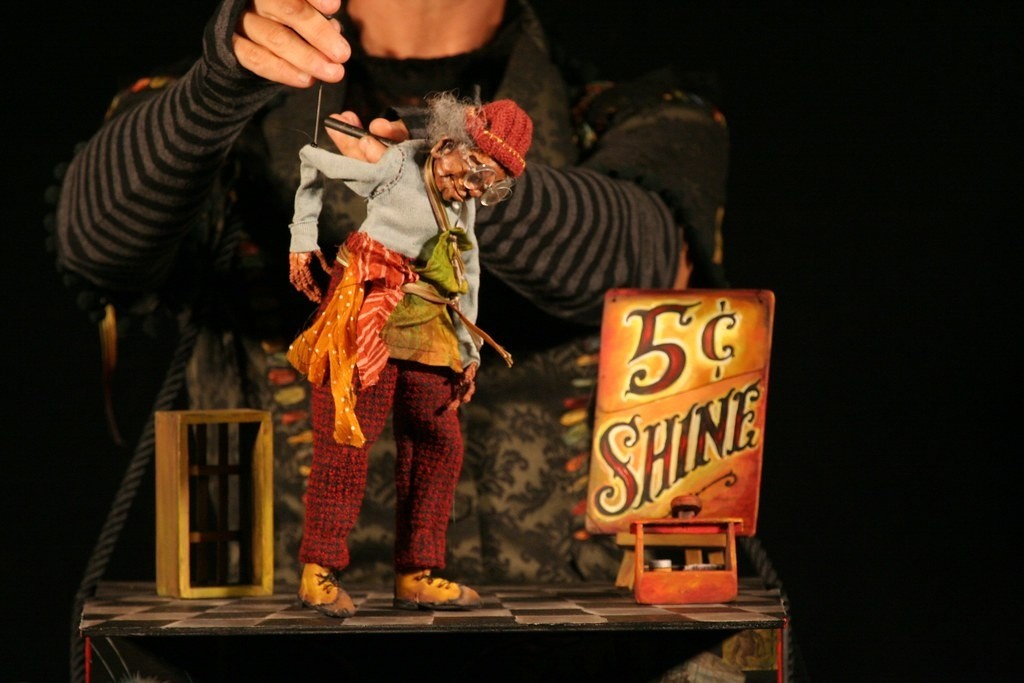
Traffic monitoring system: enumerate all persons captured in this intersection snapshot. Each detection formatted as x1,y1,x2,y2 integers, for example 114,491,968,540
56,0,728,586
289,92,534,614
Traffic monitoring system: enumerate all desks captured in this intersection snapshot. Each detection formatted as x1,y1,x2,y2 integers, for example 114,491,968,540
79,577,787,683
